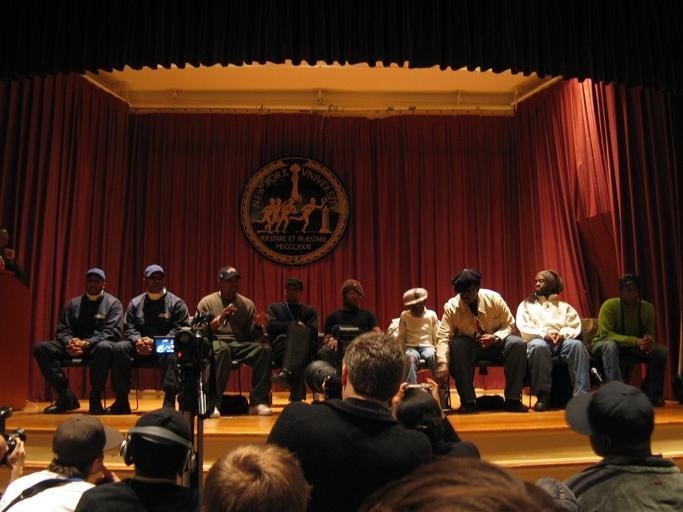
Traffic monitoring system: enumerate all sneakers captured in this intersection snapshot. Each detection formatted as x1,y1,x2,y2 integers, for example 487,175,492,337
249,404,273,416
207,404,220,418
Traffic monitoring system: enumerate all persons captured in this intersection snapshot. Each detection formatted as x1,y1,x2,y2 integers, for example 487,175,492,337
101,264,190,414
434,268,529,414
263,276,318,402
323,278,382,360
0,228,22,282
515,269,592,412
266,330,433,512
32,268,123,413
198,440,313,511
590,272,669,406
193,265,273,418
0,412,124,512
0,435,25,498
390,378,481,460
396,287,451,412
562,380,682,511
73,407,196,512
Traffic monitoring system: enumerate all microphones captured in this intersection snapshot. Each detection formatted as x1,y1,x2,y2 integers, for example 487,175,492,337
222,303,235,326
591,368,604,385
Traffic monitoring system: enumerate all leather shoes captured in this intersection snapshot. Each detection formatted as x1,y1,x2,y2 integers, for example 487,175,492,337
270,368,298,387
43,396,133,415
646,393,664,407
456,400,547,412
162,393,175,412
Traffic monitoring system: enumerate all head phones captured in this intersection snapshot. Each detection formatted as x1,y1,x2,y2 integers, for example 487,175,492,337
118,426,196,477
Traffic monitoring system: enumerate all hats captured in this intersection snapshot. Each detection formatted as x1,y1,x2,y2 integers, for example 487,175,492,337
566,381,654,446
285,279,303,291
403,267,484,306
86,268,105,280
537,267,565,294
217,266,241,281
144,264,166,279
619,274,641,291
340,279,364,296
51,415,106,467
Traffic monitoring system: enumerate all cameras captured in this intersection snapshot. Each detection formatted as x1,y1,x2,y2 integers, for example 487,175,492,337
405,384,430,397
0,407,26,464
